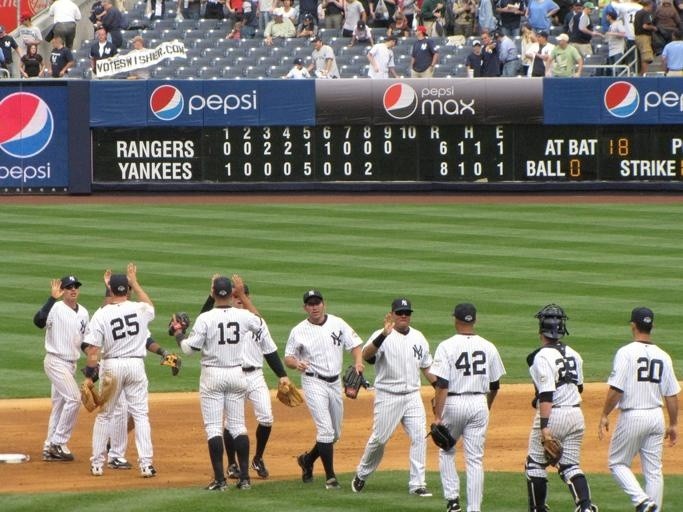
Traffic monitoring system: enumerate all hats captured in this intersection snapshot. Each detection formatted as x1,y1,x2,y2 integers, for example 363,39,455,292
629,307,653,326
417,26,430,37
522,22,570,41
584,1,595,9
391,297,413,312
214,276,250,299
60,275,81,289
472,40,482,46
302,13,314,20
357,20,365,32
271,8,284,16
309,35,321,43
110,274,129,294
293,57,306,67
303,289,323,303
451,303,476,322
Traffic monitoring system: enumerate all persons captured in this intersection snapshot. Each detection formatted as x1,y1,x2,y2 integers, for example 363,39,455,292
102,267,181,470
429,303,507,512
201,272,291,479
351,298,437,497
284,289,365,489
171,273,266,492
598,307,682,512
0,0,683,79
82,262,156,478
525,304,599,512
33,274,100,461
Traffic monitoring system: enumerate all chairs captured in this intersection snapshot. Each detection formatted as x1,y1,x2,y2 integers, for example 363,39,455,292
64,0,608,80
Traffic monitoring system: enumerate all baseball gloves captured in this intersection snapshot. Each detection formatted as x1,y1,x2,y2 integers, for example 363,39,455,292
541,435,563,467
276,380,304,407
343,366,368,397
160,352,180,374
430,422,456,450
80,363,102,412
169,313,190,336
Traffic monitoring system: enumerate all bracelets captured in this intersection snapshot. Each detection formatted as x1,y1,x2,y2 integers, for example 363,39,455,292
85,366,94,377
541,418,548,430
157,347,166,355
373,334,385,348
431,382,436,388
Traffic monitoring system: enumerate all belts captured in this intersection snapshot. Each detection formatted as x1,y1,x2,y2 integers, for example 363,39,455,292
552,403,581,408
242,366,261,373
447,392,481,396
306,372,338,383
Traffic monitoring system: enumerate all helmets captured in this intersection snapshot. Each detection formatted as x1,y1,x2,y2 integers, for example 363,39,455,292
535,302,569,340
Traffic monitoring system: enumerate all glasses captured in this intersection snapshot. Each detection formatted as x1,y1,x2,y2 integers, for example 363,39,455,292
63,284,79,290
394,310,411,317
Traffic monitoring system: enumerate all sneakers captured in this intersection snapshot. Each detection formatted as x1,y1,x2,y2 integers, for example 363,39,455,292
414,488,432,496
90,467,103,476
141,465,157,478
325,480,342,490
298,451,314,483
205,455,267,491
107,457,132,469
351,473,365,492
41,442,73,461
447,500,462,512
